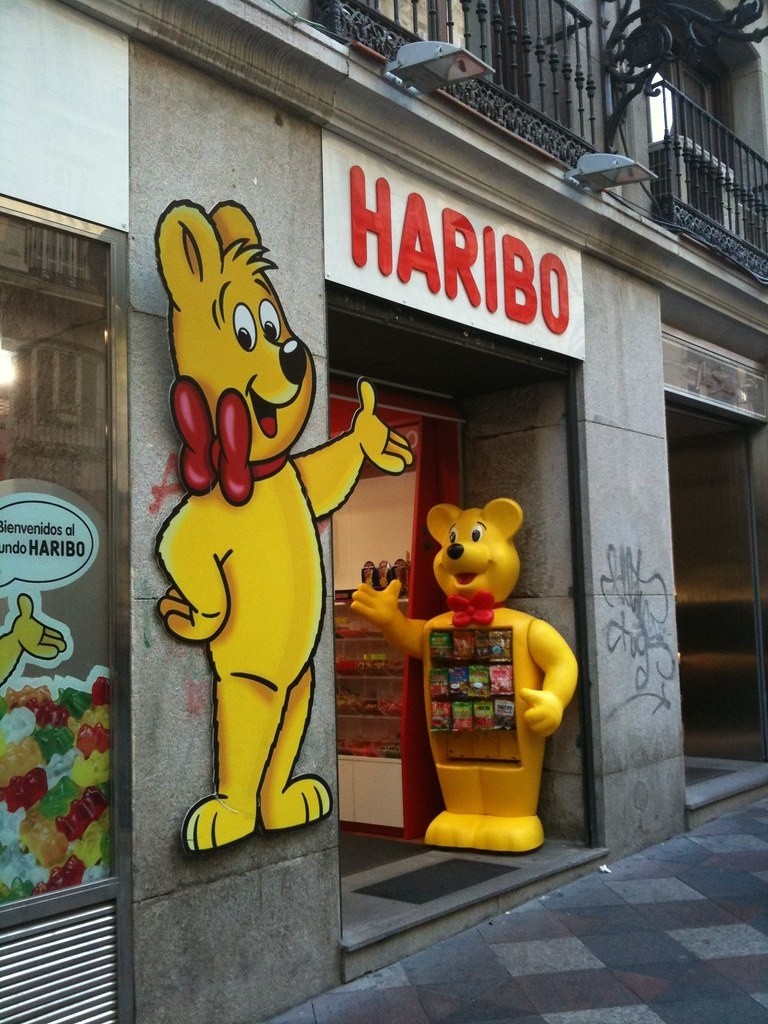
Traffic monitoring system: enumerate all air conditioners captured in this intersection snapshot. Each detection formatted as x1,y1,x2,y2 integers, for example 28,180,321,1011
648,135,745,239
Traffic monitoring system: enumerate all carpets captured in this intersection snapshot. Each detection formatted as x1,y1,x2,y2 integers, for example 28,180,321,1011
684,766,738,786
352,857,521,905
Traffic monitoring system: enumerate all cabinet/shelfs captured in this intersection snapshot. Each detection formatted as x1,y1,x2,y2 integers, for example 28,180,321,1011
329,417,462,841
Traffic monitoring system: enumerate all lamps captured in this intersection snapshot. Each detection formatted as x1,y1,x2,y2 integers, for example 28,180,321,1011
563,153,659,193
382,41,496,96
